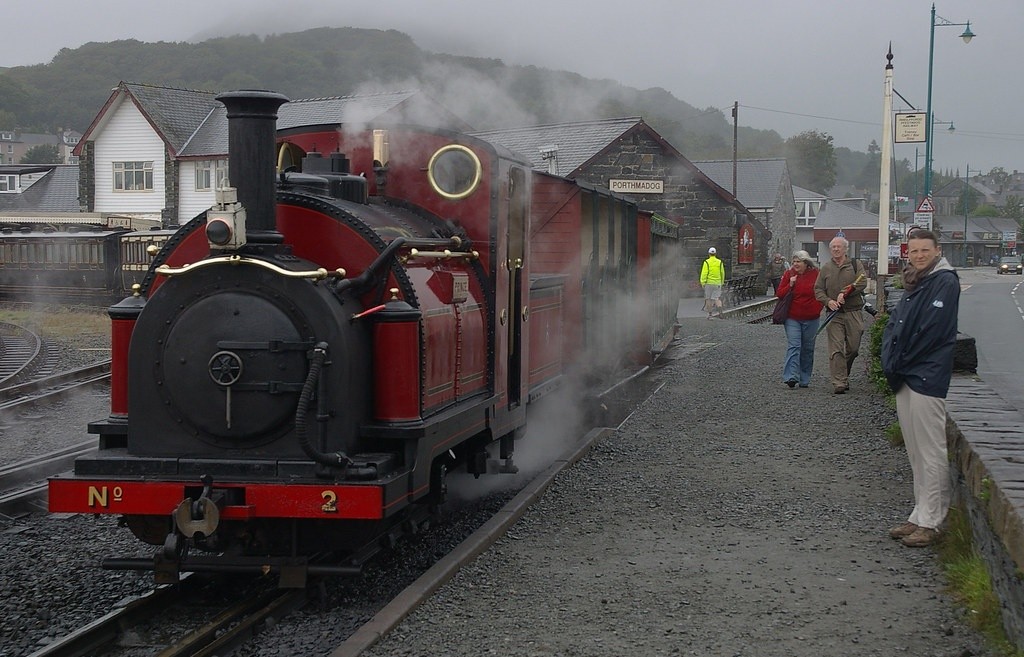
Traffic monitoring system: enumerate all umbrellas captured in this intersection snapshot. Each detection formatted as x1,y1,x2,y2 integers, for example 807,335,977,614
812,274,864,341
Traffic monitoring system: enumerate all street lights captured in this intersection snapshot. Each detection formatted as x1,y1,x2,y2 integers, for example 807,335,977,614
929,110,956,192
962,162,983,268
922,3,977,197
913,146,936,212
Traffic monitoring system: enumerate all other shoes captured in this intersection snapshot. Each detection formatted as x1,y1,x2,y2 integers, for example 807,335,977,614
718,305,725,319
890,523,918,539
706,312,714,322
834,381,849,394
900,528,944,548
787,380,796,389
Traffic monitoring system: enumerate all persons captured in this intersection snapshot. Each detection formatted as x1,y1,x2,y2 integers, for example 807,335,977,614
814,236,867,394
767,253,791,293
881,231,961,545
700,247,725,319
776,250,825,388
971,250,999,268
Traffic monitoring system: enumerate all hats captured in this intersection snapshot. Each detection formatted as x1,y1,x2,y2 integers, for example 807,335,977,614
707,246,717,255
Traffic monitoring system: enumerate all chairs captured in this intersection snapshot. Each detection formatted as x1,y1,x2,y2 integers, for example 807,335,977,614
730,278,743,305
702,279,735,310
742,274,760,300
733,276,751,302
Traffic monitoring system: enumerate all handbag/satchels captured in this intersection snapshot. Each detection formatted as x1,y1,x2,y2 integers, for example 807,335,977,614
773,289,791,323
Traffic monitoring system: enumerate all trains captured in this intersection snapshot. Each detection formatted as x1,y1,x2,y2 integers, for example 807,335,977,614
42,88,686,590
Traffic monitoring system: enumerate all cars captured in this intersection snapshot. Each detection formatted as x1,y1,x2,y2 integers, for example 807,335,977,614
996,257,1022,275
